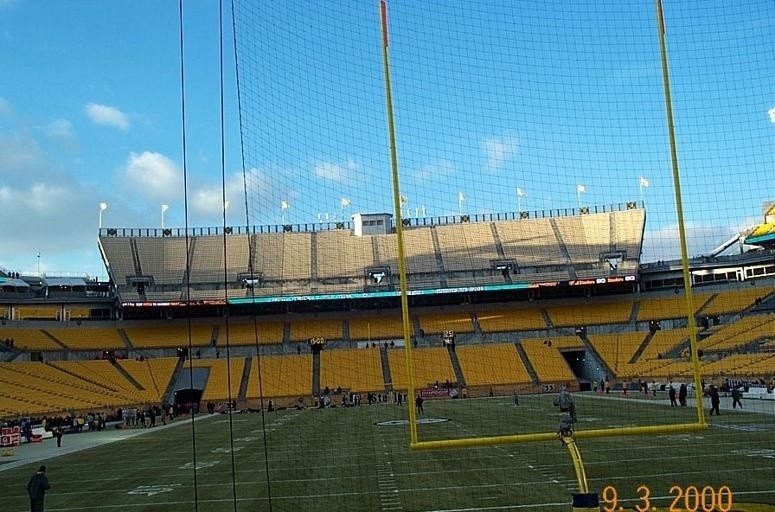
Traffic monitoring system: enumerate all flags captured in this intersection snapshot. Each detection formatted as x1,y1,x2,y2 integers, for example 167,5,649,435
578,185,585,192
282,201,289,208
460,192,465,200
517,188,525,196
343,199,351,205
162,205,168,210
101,203,107,210
641,177,648,186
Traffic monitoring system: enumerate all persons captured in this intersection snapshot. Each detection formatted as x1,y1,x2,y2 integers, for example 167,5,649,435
415,393,424,412
366,341,395,349
206,386,407,414
462,386,468,397
1,336,16,355
414,337,418,348
95,348,145,363
513,389,520,408
667,377,749,416
297,343,301,355
3,402,175,447
176,344,189,360
489,385,493,396
592,377,657,396
26,465,51,511
